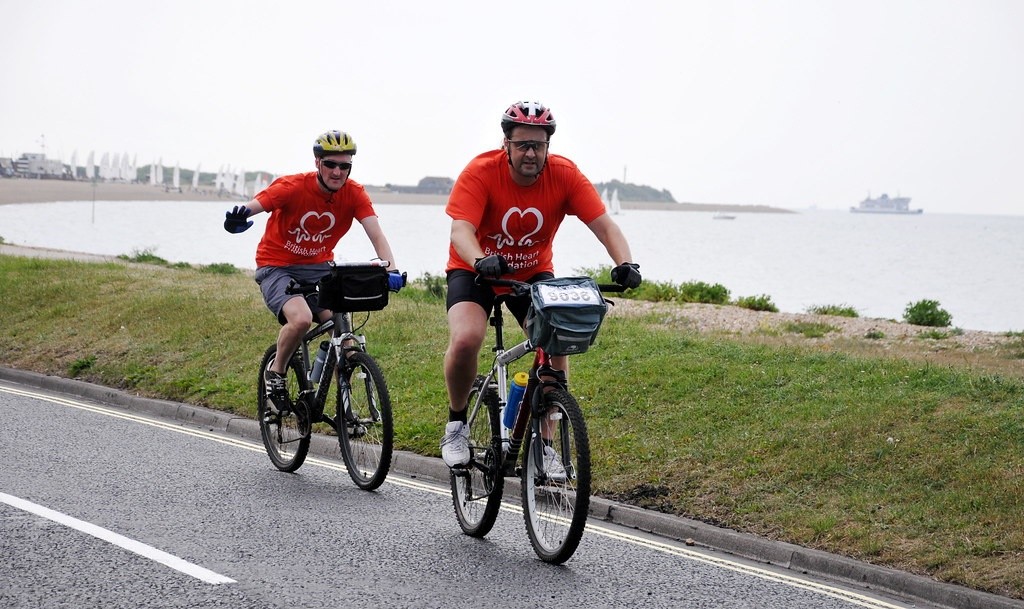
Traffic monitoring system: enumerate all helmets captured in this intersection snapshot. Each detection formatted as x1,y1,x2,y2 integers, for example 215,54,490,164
501,100,563,131
313,131,357,159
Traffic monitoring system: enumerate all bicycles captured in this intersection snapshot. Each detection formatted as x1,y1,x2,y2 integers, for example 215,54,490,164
257,271,407,491
449,275,628,566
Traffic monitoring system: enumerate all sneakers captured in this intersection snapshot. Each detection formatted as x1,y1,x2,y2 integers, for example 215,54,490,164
438,420,476,469
533,446,566,481
264,358,290,415
333,412,371,435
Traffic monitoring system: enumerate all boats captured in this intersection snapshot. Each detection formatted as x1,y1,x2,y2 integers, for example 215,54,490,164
850,194,923,214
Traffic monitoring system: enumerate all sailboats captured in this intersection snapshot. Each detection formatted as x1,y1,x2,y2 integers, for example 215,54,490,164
600,189,622,216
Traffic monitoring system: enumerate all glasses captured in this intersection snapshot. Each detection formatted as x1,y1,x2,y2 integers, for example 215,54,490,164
507,139,556,153
319,157,353,170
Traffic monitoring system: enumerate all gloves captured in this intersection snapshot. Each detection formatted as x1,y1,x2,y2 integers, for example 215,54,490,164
223,205,253,236
473,254,516,280
610,262,644,289
386,269,403,294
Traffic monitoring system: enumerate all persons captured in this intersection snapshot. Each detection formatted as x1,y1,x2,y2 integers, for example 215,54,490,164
438,99,641,480
224,130,402,436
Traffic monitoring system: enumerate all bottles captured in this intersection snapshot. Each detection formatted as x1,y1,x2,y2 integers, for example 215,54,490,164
503,372,529,430
310,341,330,384
